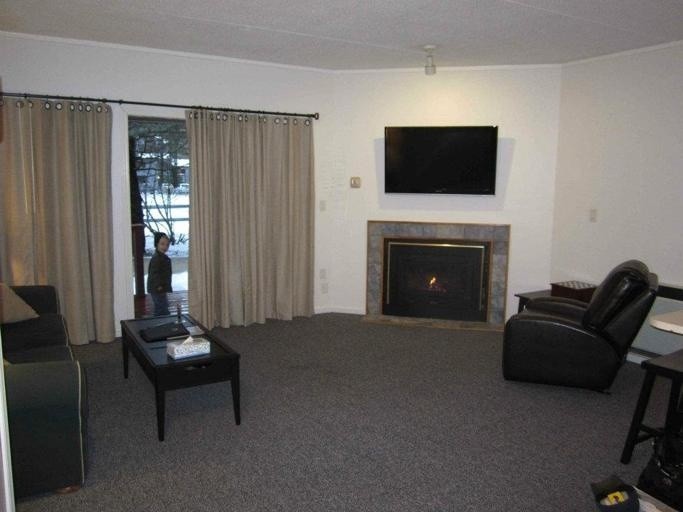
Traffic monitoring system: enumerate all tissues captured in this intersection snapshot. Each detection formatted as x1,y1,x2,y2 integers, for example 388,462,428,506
166,335,212,361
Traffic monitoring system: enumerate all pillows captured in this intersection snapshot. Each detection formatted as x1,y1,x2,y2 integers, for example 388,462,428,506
0,283,41,324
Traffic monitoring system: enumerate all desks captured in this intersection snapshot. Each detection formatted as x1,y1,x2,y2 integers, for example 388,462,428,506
648,309,682,336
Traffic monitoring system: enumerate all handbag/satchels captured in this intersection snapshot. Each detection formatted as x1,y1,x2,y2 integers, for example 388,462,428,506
636,452,683,512
592,475,639,512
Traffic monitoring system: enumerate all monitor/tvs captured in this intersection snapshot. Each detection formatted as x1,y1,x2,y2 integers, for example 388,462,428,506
385,126,495,194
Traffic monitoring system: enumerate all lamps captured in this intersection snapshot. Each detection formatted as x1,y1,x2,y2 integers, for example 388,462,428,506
424,41,435,75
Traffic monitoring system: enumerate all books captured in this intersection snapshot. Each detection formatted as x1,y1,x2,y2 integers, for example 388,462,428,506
165,326,205,340
139,321,190,343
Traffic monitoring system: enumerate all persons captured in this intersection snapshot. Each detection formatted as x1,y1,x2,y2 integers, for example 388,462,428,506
146,232,172,316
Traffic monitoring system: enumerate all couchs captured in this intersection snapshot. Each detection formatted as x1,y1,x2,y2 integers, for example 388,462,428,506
1,286,88,499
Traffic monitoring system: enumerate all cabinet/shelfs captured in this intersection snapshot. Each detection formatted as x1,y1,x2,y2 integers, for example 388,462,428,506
549,279,597,304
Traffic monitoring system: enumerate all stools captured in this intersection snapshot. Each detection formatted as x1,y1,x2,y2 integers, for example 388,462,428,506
512,289,550,316
617,349,682,466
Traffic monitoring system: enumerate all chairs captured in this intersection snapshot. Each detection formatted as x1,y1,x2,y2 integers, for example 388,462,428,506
502,257,658,394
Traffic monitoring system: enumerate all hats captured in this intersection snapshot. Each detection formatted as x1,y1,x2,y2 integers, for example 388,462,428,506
154,233,165,247
